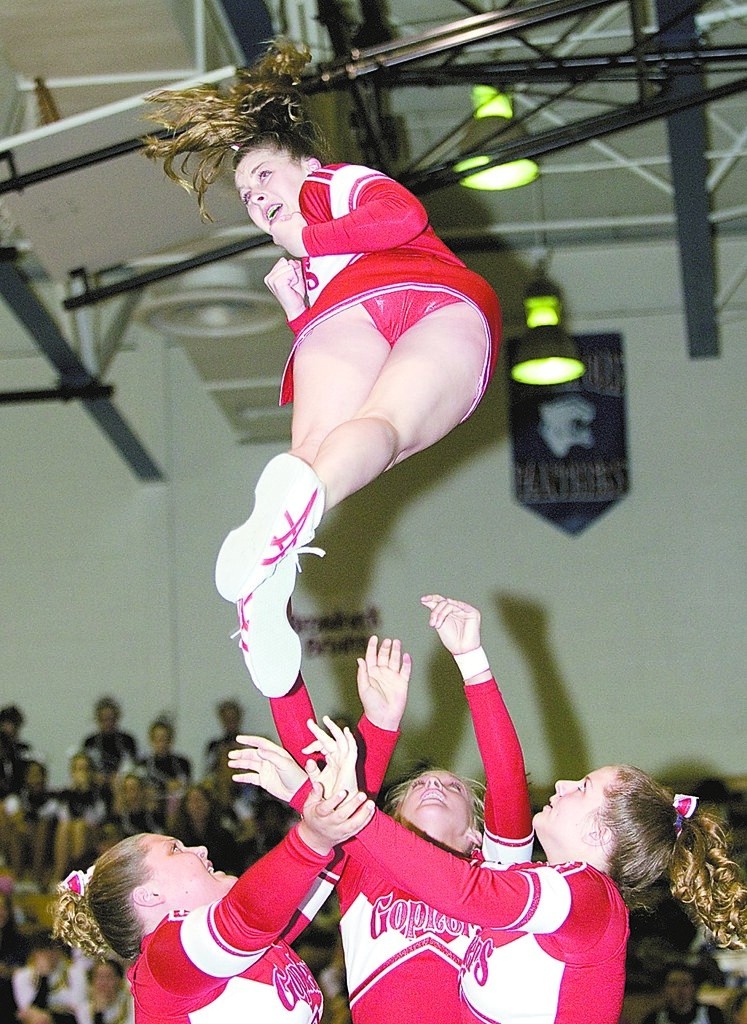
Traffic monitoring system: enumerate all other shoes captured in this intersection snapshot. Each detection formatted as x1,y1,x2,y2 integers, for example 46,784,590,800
230,546,330,699
214,453,325,605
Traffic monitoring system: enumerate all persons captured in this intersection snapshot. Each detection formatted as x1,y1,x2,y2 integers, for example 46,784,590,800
144,34,502,698
0,595,747,1024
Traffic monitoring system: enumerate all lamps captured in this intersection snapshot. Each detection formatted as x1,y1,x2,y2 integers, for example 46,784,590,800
508,260,588,385
448,84,539,191
134,264,286,338
